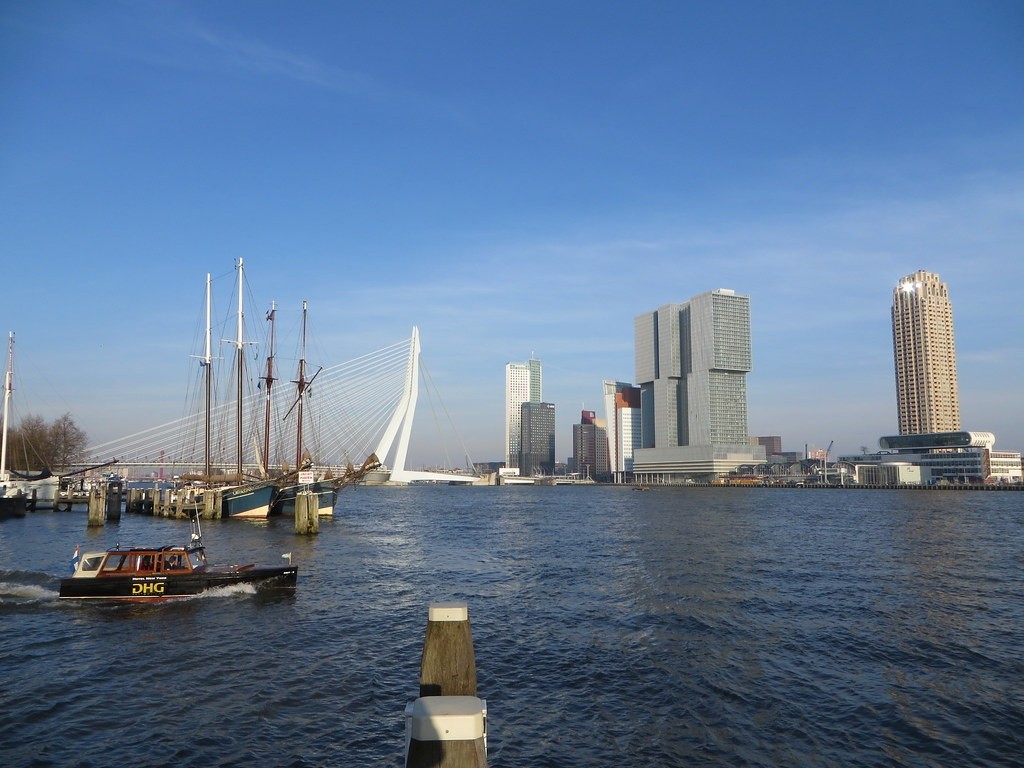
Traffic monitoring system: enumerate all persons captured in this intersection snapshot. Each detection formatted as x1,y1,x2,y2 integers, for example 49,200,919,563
138,554,182,571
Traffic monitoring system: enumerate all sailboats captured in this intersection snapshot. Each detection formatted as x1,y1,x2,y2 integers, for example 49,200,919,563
0,254,382,520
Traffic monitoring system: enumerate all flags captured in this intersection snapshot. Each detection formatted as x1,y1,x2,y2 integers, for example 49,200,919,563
68,543,80,576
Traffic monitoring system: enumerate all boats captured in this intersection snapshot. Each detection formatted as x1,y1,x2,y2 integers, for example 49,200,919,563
58,503,298,609
